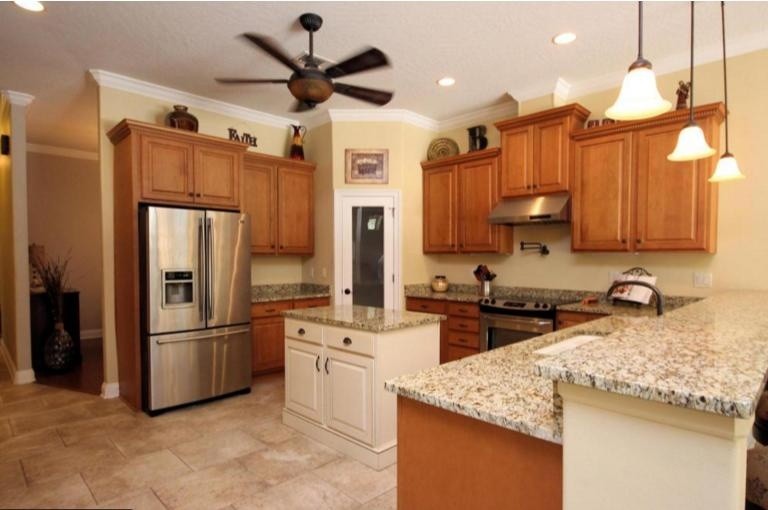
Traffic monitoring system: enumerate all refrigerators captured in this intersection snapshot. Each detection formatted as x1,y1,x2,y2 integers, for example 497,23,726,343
145,206,252,411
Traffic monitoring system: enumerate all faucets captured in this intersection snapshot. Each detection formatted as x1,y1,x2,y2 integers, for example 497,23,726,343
607,280,664,316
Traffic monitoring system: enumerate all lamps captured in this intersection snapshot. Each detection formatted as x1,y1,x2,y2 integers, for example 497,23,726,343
604,0,671,119
707,1,743,179
667,1,715,161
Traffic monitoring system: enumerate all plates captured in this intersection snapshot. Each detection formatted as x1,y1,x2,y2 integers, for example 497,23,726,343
427,138,460,161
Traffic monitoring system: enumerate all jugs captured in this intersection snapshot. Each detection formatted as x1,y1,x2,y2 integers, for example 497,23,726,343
467,126,488,153
290,124,307,160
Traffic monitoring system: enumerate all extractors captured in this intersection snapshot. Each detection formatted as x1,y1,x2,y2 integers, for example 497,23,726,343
487,192,572,225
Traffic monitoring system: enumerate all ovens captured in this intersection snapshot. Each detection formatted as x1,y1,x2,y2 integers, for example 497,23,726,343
480,312,553,355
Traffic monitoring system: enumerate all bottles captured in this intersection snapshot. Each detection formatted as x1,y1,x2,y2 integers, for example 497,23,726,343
170,105,198,133
431,276,449,291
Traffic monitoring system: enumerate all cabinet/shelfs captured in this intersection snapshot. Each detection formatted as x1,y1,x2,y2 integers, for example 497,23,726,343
282,317,441,452
109,118,331,377
30,286,83,368
406,101,732,363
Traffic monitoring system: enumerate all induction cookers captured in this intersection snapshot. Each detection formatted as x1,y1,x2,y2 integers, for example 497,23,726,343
479,294,577,320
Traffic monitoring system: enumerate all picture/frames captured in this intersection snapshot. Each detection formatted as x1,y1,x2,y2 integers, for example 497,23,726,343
345,148,389,183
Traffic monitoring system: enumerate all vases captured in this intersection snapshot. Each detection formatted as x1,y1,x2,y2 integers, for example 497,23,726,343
47,324,74,370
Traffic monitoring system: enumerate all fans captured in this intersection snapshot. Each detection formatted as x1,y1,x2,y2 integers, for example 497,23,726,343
215,14,392,110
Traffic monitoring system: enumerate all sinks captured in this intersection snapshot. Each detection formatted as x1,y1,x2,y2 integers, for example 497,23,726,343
532,334,604,356
